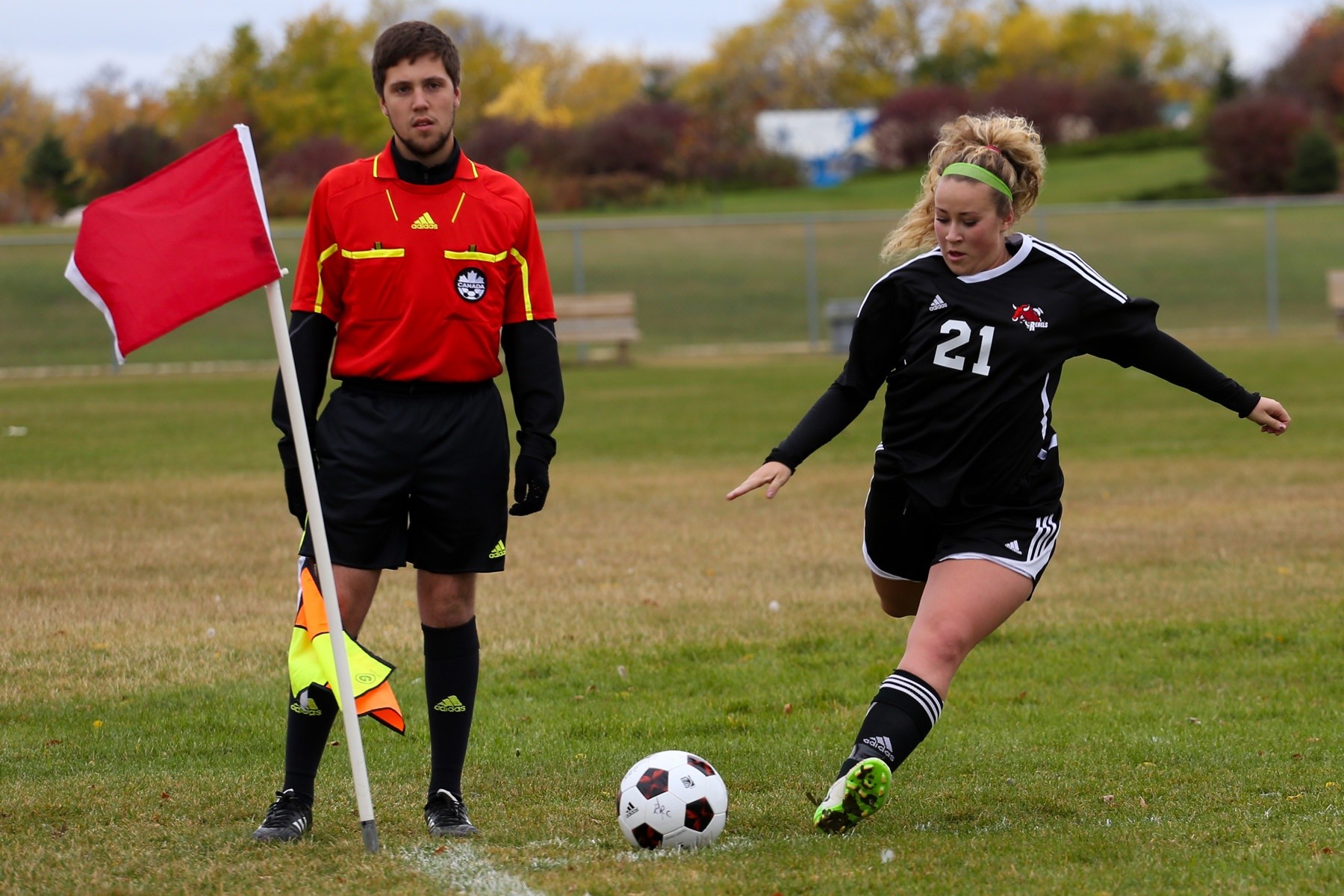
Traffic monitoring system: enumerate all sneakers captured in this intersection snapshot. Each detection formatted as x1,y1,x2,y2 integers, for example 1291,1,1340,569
251,792,315,843
813,756,892,834
422,790,477,838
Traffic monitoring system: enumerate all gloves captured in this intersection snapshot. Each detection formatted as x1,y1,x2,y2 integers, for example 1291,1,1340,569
508,430,556,516
276,431,307,531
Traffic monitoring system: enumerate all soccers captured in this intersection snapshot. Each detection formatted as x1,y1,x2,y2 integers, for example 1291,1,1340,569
615,750,730,853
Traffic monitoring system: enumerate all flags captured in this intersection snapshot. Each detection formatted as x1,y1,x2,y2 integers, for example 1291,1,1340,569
64,128,281,368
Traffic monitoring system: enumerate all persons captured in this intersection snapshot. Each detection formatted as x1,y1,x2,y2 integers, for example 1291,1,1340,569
729,109,1290,831
254,20,566,841
